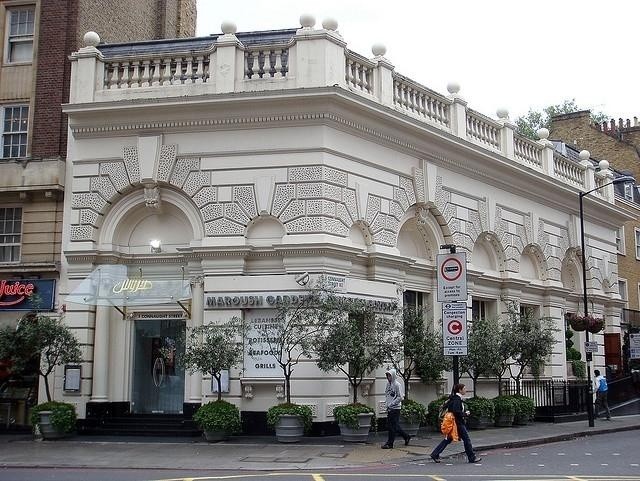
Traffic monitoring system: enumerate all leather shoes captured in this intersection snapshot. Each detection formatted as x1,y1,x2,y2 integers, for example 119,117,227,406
430,454,440,462
472,457,481,463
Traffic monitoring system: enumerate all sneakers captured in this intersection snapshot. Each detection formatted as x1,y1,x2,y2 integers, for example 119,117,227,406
381,444,393,448
405,435,411,445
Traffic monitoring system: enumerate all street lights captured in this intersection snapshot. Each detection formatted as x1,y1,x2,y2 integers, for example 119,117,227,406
576,174,635,428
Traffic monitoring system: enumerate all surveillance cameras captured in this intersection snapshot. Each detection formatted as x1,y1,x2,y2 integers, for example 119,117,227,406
296,272,308,284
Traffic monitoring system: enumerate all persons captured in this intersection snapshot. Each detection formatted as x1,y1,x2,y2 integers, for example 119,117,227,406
593,370,611,418
430,384,482,463
381,369,411,448
